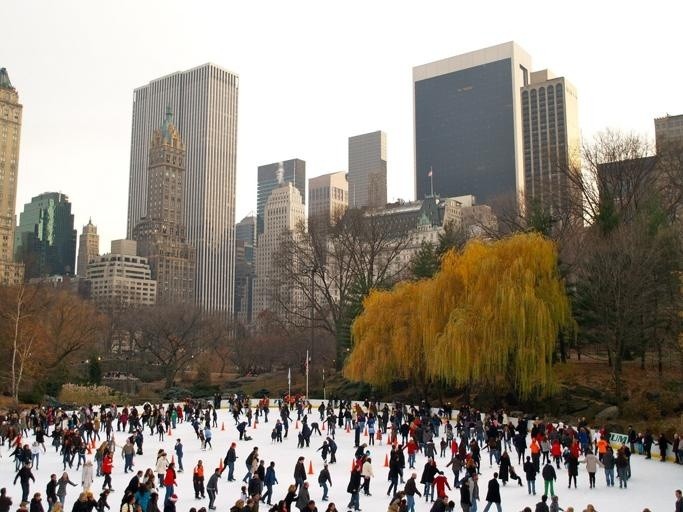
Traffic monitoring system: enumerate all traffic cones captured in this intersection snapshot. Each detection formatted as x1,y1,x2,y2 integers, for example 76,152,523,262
91,439,95,449
168,427,171,435
351,460,355,470
383,455,388,467
307,461,313,474
293,420,397,451
87,444,91,454
253,421,256,429
221,422,224,430
218,458,222,471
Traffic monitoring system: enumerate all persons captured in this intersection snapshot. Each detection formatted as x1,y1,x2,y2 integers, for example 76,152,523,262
1,390,681,511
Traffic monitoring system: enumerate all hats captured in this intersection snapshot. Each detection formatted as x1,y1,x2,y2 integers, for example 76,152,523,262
170,495,178,502
